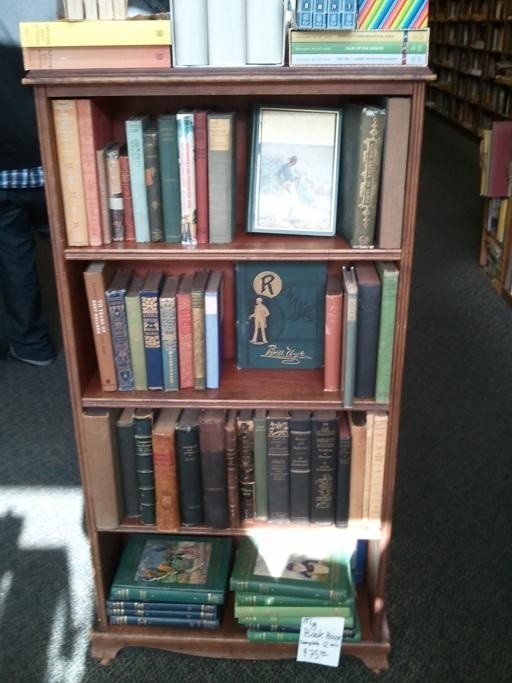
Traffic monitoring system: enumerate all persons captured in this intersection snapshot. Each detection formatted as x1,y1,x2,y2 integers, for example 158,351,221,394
0,44,51,366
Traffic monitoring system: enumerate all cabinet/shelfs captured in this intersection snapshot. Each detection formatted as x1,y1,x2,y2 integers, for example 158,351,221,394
479,197,511,304
21,69,437,670
426,1,512,135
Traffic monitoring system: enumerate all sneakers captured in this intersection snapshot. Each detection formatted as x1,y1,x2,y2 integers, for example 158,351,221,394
1,343,53,367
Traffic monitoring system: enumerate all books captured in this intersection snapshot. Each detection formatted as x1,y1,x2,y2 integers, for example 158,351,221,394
170,0,286,67
56,0,128,23
431,0,512,295
106,534,361,644
52,98,387,249
84,260,399,408
82,407,389,532
